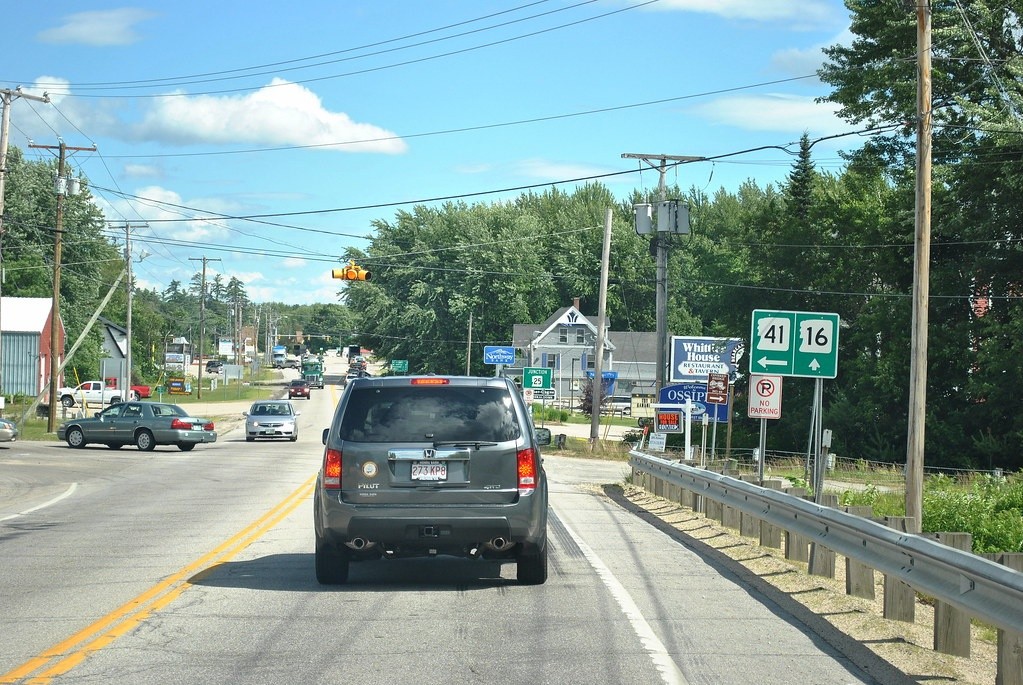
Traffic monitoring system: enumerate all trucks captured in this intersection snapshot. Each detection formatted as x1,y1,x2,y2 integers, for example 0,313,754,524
297,361,326,389
272,346,287,367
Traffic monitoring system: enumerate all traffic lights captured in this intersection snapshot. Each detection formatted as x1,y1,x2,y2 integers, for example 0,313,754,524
346,265,359,280
332,267,347,281
357,266,372,282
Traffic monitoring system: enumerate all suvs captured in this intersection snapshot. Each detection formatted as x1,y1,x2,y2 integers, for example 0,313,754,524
311,373,552,586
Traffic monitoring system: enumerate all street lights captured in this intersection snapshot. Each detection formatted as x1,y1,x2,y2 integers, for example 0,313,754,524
125,248,153,401
530,330,543,368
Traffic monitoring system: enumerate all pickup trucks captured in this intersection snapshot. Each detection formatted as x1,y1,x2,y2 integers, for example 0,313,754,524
57,381,136,408
83,377,150,401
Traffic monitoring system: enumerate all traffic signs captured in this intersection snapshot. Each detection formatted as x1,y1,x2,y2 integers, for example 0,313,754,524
705,371,729,406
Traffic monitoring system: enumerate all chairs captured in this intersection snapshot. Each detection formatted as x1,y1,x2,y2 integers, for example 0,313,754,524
275,405,287,414
374,402,412,438
256,406,267,415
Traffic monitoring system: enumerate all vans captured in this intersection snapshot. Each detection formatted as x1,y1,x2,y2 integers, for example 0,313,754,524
600,396,632,416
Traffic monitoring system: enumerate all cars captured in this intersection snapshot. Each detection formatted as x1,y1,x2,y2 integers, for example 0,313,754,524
0,418,19,442
287,379,310,399
276,359,301,369
343,343,371,390
57,402,217,452
242,400,300,442
205,360,223,374
